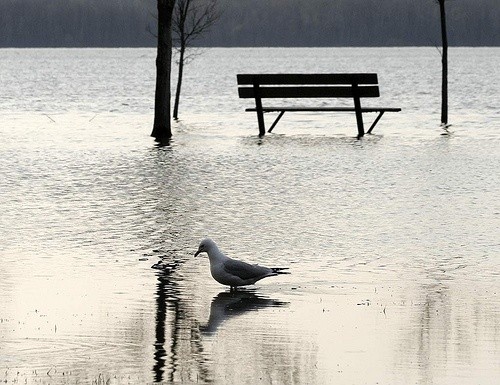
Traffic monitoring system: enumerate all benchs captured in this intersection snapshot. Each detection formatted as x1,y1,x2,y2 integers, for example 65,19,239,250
236,73,401,137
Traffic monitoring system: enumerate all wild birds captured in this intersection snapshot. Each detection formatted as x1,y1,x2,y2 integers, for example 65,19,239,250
200,290,290,341
193,238,292,290
